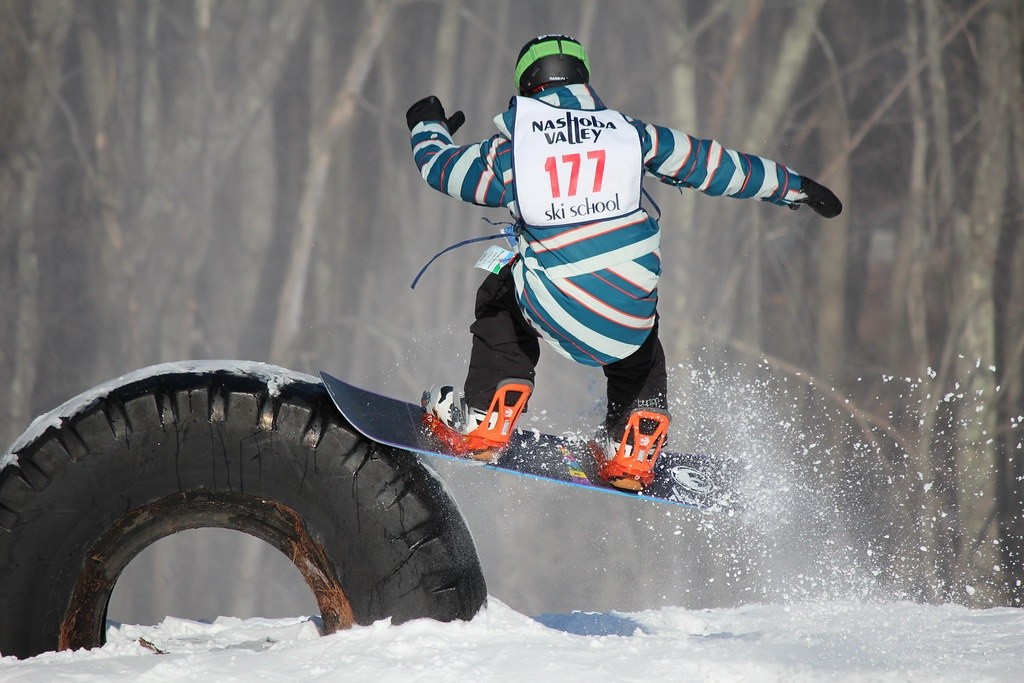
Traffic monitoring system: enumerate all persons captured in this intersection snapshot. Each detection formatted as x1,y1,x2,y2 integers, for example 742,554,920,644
406,34,842,463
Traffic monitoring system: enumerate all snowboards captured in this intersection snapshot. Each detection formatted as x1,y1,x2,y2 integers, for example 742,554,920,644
316,367,749,511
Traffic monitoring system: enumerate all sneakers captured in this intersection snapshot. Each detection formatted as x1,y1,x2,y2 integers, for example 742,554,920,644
593,421,656,462
430,385,510,436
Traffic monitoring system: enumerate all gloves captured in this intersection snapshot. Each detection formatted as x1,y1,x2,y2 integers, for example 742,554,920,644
790,178,842,219
406,96,465,136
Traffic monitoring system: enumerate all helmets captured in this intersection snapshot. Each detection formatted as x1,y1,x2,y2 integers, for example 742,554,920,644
515,34,590,97
